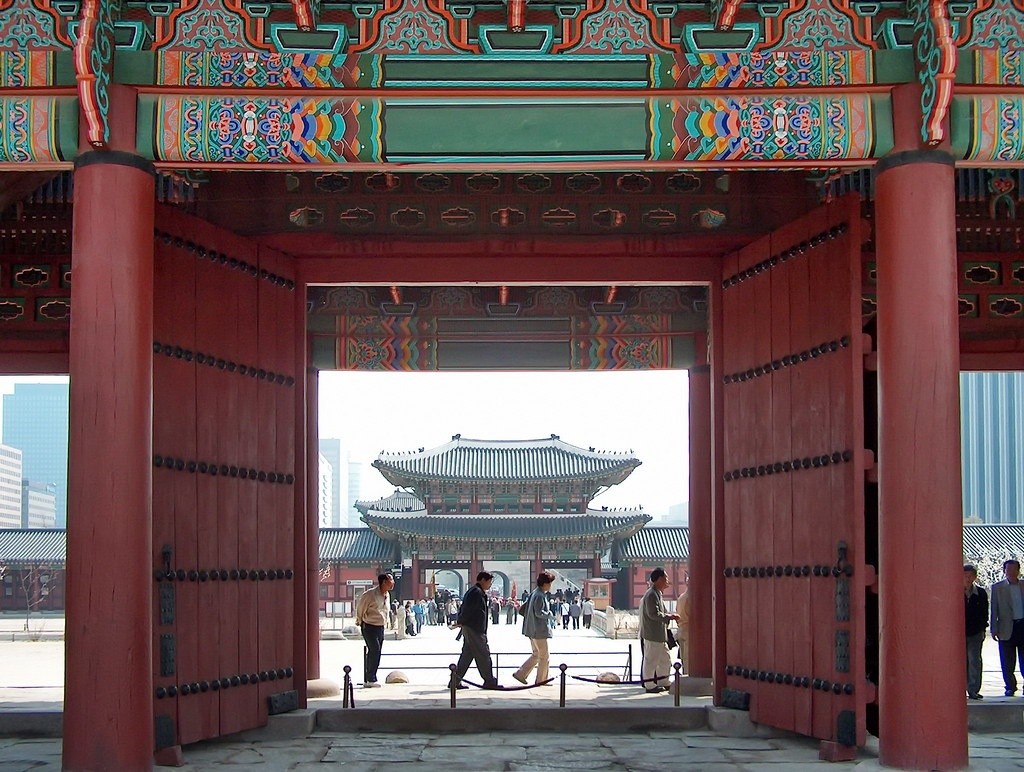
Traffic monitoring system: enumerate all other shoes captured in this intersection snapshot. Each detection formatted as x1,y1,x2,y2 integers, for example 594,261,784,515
364,680,381,687
513,673,528,684
969,691,983,699
483,682,504,689
448,683,468,689
1005,689,1013,696
411,633,417,636
542,683,554,687
646,685,666,693
660,684,672,692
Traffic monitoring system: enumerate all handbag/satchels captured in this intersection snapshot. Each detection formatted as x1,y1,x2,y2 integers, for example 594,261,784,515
666,628,679,650
519,600,527,616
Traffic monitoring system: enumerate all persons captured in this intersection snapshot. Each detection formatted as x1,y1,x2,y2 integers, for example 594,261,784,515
676,575,688,674
448,572,505,690
513,572,555,686
389,587,595,636
991,560,1024,697
963,565,989,699
639,567,680,693
356,572,395,688
